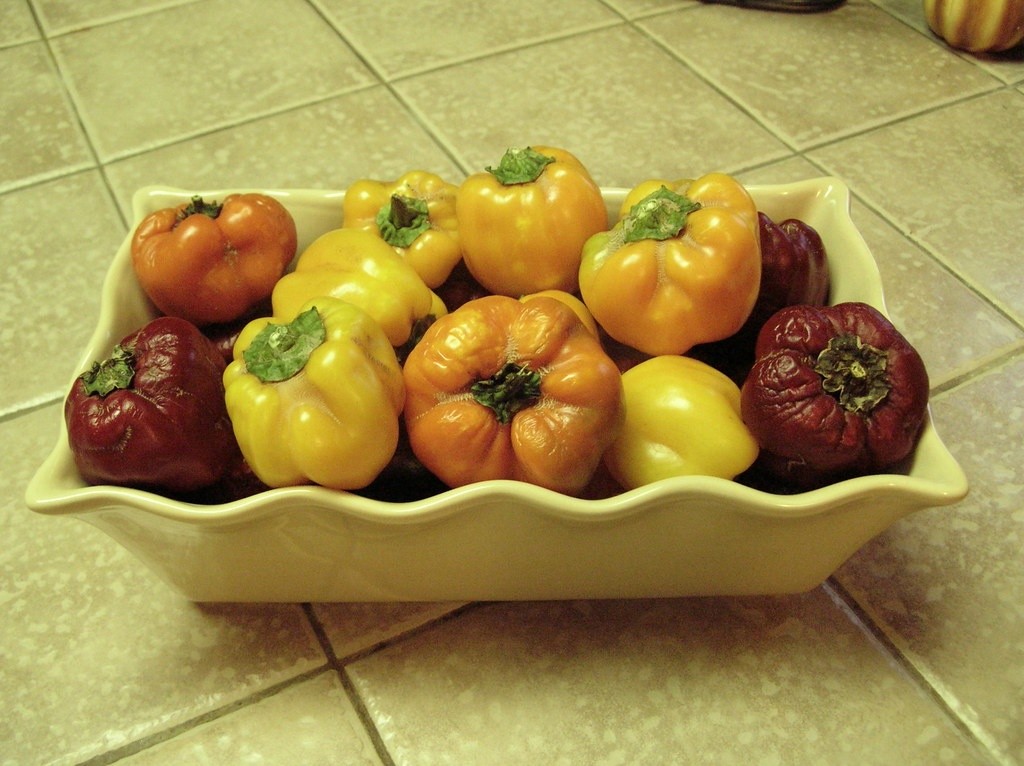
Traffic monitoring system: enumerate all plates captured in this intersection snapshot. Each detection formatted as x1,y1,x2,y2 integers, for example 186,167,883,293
22,174,969,605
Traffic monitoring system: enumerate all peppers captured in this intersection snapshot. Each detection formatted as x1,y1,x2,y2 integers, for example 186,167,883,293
63,146,930,506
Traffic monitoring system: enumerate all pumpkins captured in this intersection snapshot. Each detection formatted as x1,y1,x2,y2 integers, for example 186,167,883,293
921,0,1024,56
